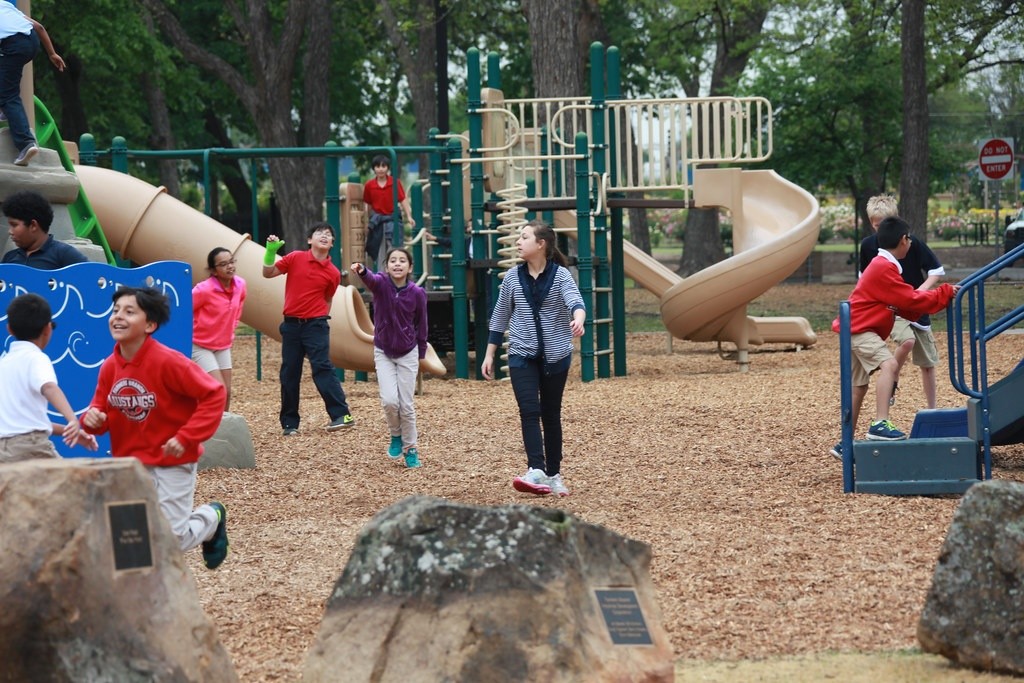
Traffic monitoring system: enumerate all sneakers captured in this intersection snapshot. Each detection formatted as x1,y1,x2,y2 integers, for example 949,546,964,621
513,466,551,494
549,473,569,498
889,381,897,406
202,500,229,570
282,428,299,435
13,142,39,166
386,436,403,459
829,441,843,462
326,414,356,432
403,448,422,468
866,420,906,441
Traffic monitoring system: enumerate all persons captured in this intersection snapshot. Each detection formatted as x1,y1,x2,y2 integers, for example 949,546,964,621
192,247,247,412
350,247,428,469
362,154,416,261
263,222,355,436
858,196,945,408
79,285,230,571
481,223,587,497
0,191,90,270
426,219,474,258
0,0,66,166
829,216,963,461
0,294,99,462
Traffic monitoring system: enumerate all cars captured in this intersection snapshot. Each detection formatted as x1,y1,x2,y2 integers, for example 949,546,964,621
1004,207,1024,267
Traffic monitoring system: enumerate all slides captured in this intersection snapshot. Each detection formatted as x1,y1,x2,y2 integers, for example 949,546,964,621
74,143,448,394
660,169,821,346
537,209,817,345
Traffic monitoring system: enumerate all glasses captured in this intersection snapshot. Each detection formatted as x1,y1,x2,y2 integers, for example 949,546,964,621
211,260,237,268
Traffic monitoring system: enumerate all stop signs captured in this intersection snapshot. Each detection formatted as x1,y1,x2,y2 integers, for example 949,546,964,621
977,137,1015,181
